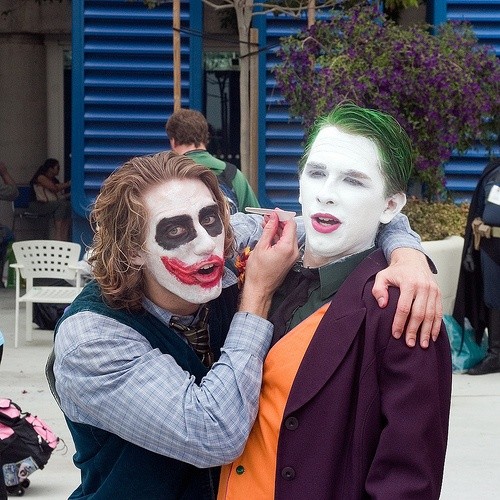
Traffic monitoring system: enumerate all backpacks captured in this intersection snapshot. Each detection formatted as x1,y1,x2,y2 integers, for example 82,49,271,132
216,162,239,215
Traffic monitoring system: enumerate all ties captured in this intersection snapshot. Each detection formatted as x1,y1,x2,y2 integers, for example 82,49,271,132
268,268,319,348
170,306,213,369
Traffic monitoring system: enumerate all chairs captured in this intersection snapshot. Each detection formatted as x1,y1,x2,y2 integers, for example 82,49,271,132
9,240,93,348
8,184,42,233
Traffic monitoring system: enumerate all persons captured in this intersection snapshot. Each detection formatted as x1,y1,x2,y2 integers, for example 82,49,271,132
31,159,70,241
166,109,261,213
216,101,453,500
45,150,445,500
453,162,499,376
0,160,19,288
0,330,8,500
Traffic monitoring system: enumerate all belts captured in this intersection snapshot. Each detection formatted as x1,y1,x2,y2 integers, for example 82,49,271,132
489,227,500,237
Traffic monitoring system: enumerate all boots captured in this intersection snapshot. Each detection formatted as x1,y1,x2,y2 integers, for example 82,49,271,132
468,310,500,375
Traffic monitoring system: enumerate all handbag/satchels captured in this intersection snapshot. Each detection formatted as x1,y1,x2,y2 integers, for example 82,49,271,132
33,183,67,202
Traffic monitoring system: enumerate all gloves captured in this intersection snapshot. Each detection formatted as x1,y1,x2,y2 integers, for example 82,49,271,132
462,248,475,273
